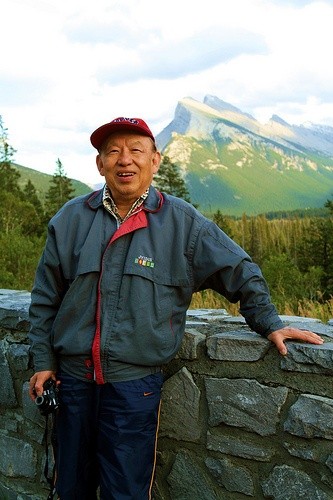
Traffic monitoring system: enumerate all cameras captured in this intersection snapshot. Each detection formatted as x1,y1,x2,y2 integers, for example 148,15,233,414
33,377,62,416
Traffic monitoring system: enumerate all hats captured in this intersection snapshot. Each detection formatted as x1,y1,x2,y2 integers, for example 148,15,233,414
90,116,158,153
